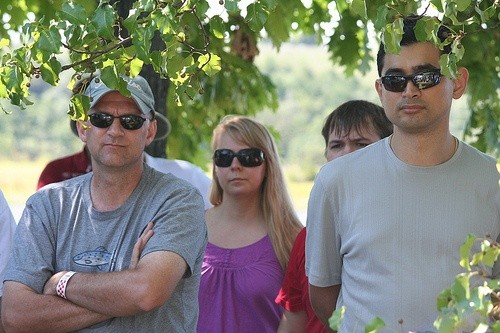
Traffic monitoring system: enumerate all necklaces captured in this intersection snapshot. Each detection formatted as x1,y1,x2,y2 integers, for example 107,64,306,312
389,135,457,149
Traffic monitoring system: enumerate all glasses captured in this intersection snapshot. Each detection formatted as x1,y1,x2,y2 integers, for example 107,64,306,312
213,147,266,168
381,68,443,93
89,111,148,131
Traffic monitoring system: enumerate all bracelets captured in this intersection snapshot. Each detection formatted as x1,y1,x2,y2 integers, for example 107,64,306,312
56,271,77,299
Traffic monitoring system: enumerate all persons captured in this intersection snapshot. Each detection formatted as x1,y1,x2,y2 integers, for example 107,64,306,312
275,101,394,333
0,186,18,333
36,77,215,212
1,75,209,333
196,114,304,333
305,14,500,333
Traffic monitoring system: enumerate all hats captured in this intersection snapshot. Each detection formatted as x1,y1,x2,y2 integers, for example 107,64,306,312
84,73,155,115
